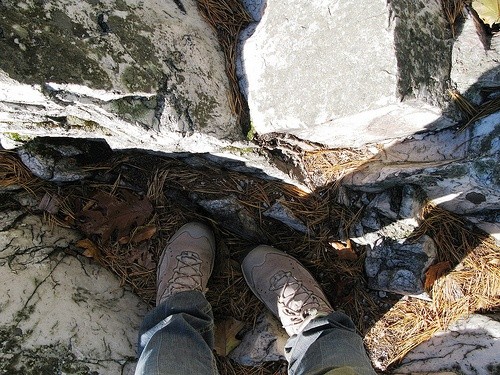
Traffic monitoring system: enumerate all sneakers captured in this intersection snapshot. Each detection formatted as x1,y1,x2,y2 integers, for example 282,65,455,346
156,220,215,307
237,243,336,338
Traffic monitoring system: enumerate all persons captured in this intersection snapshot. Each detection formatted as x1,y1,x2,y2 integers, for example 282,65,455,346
132,221,378,375
